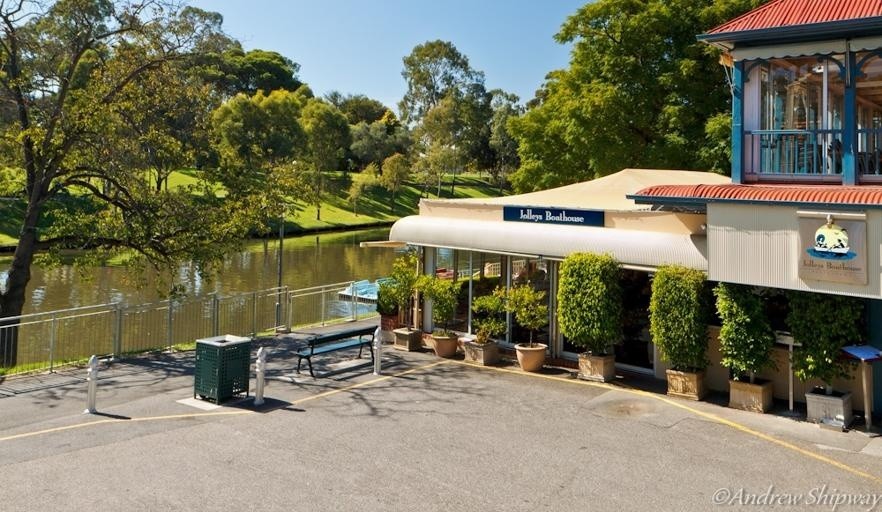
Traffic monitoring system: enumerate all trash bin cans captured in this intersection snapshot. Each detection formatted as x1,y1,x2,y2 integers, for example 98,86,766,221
194,334,252,406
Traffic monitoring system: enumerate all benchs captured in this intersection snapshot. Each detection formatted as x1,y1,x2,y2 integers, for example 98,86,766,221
289,325,378,377
